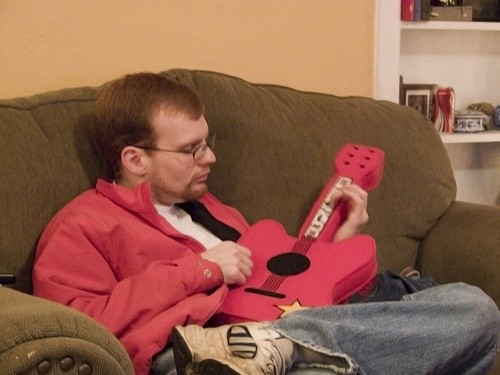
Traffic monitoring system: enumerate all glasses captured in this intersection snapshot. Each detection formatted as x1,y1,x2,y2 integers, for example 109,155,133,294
118,132,216,161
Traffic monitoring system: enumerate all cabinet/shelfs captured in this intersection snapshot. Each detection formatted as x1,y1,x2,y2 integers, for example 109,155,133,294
375,0,500,209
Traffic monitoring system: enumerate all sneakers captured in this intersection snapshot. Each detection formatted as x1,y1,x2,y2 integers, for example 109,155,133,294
171,320,294,375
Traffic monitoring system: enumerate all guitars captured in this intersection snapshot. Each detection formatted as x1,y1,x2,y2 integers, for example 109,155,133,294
217,142,386,328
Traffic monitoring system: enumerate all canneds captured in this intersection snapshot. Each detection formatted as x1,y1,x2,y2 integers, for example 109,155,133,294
431,87,455,134
401,0,414,21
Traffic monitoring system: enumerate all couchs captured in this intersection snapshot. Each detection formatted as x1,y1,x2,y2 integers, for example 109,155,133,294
0,68,500,375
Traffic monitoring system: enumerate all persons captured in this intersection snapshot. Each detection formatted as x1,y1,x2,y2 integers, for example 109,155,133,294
31,73,500,375
